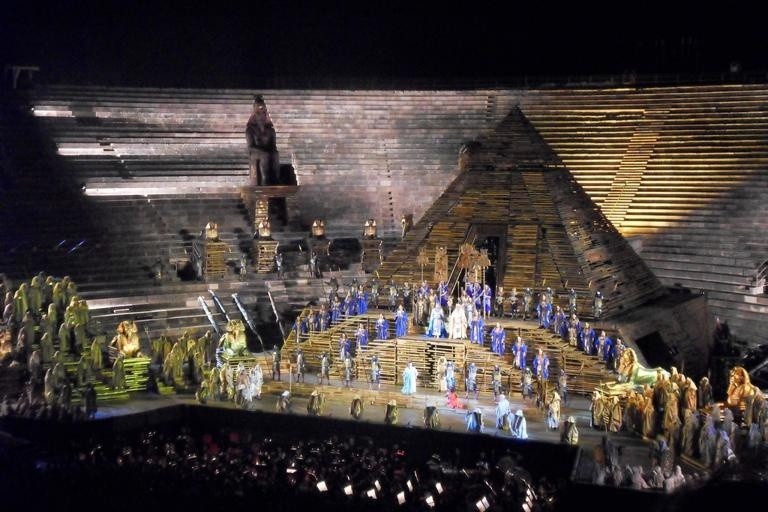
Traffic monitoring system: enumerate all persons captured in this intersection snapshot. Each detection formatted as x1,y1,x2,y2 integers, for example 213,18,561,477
0,269,264,419
591,348,767,493
272,277,626,446
70,426,567,512
246,95,281,184
